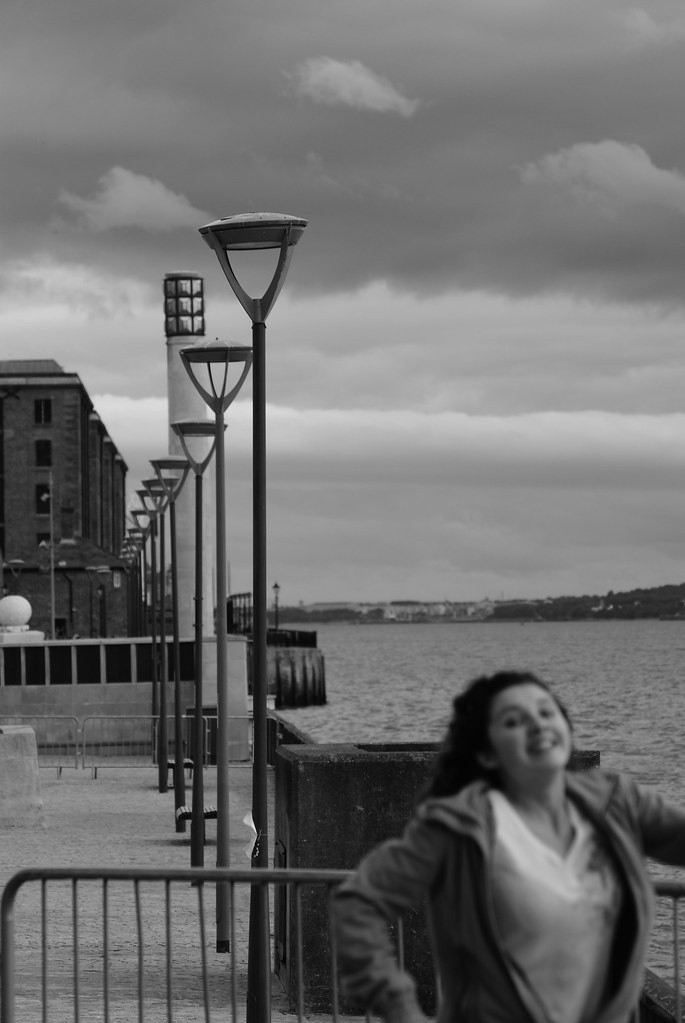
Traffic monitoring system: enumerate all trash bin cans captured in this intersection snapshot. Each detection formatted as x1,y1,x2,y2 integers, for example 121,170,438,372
186,706,217,762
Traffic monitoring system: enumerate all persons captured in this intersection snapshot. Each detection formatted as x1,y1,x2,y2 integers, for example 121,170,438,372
331,669,685,1023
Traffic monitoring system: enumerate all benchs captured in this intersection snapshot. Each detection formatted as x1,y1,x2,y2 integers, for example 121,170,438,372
175,803,217,840
167,757,193,769
